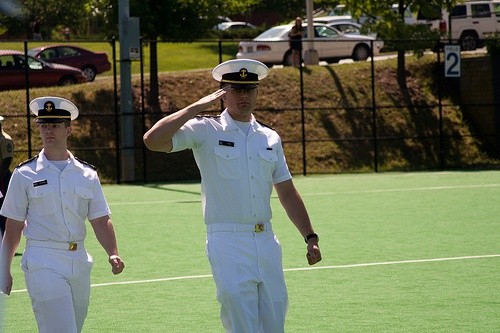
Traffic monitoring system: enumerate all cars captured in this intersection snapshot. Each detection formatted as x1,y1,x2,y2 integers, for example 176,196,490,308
0,50,88,90
235,23,381,69
304,0,500,51
199,16,259,39
27,43,112,83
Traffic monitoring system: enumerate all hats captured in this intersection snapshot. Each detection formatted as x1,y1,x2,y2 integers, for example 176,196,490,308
29,96,79,123
211,58,271,90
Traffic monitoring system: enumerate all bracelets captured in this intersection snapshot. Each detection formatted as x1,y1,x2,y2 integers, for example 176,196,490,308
305,233,319,243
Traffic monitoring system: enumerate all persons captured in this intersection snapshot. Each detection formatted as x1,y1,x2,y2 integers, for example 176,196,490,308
143,59,322,333
0,97,124,333
0,115,18,256
289,17,304,67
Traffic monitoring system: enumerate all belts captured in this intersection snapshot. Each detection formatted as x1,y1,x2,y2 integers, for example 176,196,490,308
207,222,273,232
26,239,86,250
